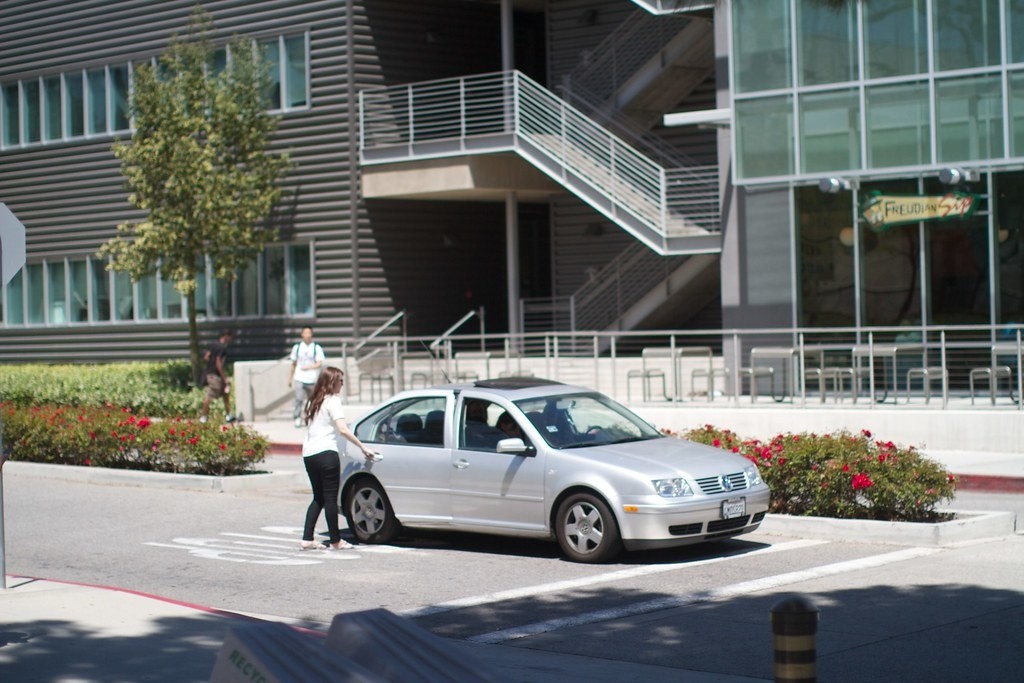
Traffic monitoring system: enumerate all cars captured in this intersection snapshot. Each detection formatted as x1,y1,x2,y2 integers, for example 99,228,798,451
337,376,770,563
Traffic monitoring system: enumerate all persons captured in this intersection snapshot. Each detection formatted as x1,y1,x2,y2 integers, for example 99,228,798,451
299,367,376,549
199,332,235,423
495,408,597,449
288,327,326,424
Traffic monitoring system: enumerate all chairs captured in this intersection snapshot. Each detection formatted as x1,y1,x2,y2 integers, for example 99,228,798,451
468,402,508,448
426,410,445,444
398,414,425,443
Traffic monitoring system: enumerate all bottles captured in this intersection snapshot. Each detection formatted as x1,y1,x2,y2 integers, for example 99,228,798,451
224,377,233,393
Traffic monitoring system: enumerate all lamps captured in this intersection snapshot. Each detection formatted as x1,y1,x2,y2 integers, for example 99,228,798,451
938,167,980,187
443,233,460,247
818,176,859,194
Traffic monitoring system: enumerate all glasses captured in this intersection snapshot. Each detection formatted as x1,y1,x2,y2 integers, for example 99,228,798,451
334,380,343,386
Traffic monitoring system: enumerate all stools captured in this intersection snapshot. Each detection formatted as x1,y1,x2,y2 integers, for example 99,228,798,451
498,370,536,377
410,370,446,390
628,368,666,401
452,370,479,384
907,366,948,405
735,367,776,404
692,367,731,404
833,367,871,405
359,372,396,405
969,365,1015,407
803,365,844,404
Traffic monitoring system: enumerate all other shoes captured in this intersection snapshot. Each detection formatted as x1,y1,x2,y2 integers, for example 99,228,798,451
226,418,236,423
329,539,353,550
294,421,302,427
300,540,326,550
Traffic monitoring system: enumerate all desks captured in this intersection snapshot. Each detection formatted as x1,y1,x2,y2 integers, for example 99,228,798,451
374,352,434,396
992,345,1024,406
641,346,714,402
454,350,521,381
851,346,926,405
750,347,826,403
